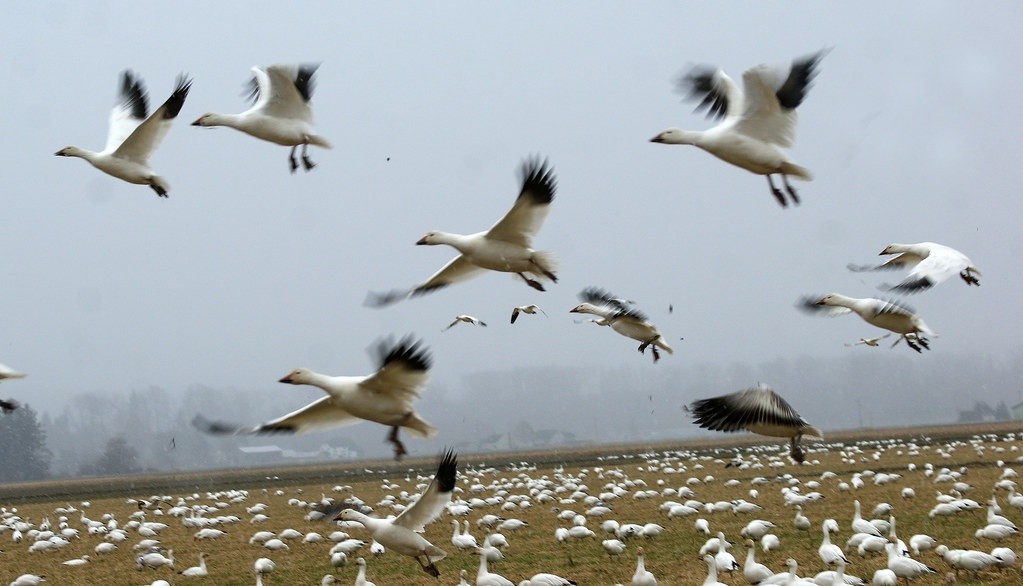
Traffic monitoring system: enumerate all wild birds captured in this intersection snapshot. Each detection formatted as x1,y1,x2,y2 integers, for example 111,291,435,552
569,288,672,363
251,332,438,458
686,380,823,465
54,68,194,198
446,315,487,329
873,244,983,294
0,430,1023,586
798,291,939,353
0,364,26,414
190,59,331,173
649,45,830,208
510,304,549,323
406,151,562,298
